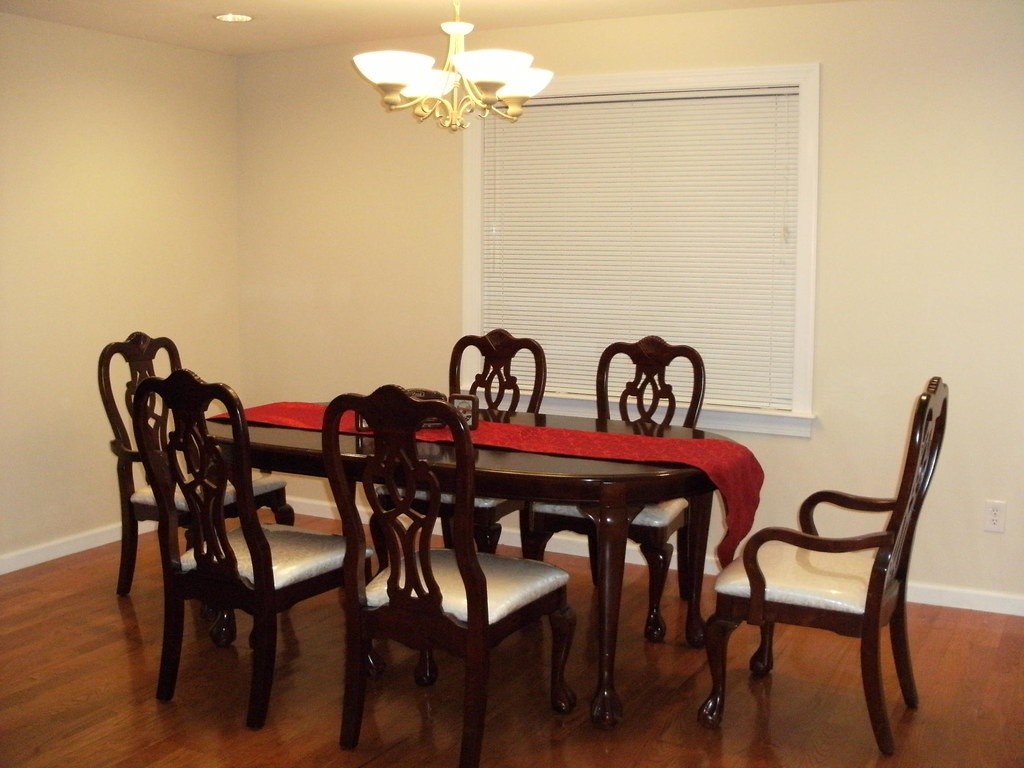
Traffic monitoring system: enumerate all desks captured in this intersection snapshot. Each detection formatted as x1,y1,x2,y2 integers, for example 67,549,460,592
184,403,748,731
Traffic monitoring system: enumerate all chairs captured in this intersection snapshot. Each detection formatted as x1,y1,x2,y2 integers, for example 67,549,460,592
96,329,950,767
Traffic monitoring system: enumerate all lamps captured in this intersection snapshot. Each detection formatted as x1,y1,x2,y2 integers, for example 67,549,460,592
349,1,552,132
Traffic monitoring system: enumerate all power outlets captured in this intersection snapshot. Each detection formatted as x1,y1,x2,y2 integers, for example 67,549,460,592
982,500,1007,533
